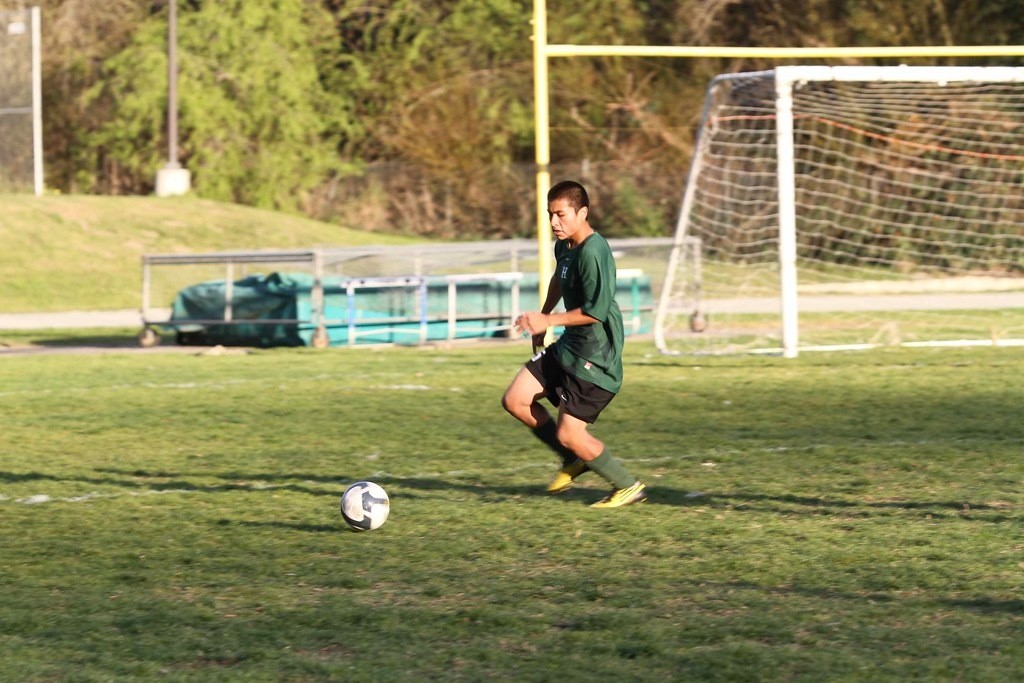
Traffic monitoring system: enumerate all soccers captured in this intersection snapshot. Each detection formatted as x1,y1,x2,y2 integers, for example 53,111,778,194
340,480,390,533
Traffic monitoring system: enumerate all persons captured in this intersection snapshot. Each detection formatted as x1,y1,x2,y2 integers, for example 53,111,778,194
502,181,649,508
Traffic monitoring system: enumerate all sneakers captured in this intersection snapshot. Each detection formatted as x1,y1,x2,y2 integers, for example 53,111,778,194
546,457,591,494
587,477,648,507
